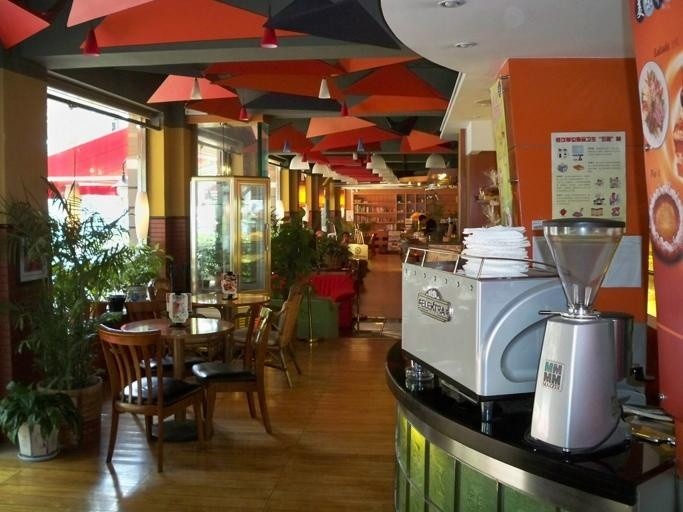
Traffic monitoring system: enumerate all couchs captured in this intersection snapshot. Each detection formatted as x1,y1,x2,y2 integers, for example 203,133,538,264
271,272,356,329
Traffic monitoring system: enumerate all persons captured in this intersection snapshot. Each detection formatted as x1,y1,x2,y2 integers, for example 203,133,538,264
419,214,437,237
340,231,349,244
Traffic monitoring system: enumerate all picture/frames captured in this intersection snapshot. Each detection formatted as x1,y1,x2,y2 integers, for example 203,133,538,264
16,231,52,285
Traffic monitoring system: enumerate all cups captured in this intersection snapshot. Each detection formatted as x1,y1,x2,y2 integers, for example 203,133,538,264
404,352,435,393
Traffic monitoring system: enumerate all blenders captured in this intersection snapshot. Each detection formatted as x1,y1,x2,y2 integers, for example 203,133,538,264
531,217,634,458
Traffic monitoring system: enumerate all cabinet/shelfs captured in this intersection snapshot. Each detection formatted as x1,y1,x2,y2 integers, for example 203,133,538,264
352,194,397,247
397,192,437,253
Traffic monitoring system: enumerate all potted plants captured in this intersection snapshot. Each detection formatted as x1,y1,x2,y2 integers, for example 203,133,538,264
315,235,347,271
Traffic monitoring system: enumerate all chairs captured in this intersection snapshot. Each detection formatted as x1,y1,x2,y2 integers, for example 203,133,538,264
98,324,206,473
191,305,274,437
233,280,309,390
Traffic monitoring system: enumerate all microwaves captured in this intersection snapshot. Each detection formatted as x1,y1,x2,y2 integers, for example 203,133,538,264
399,259,573,424
